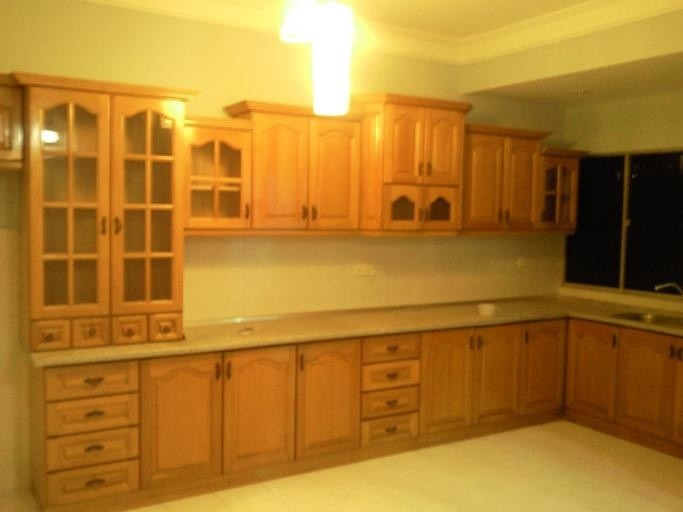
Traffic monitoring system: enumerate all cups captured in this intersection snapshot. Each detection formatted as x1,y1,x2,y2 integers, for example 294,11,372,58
477,304,496,318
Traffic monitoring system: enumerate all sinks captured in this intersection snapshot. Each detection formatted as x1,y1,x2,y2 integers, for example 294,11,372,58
612,312,682,328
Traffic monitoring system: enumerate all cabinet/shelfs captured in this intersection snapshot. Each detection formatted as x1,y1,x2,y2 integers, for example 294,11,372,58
224,102,360,237
140,344,297,507
565,318,617,438
419,321,519,455
296,338,361,477
519,319,565,432
0,76,24,172
361,332,419,464
465,130,540,237
539,154,580,235
617,324,682,462
16,73,189,350
349,93,474,239
27,358,140,512
183,121,251,235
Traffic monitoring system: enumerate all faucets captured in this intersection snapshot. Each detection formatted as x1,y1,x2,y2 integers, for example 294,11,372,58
655,282,683,294
